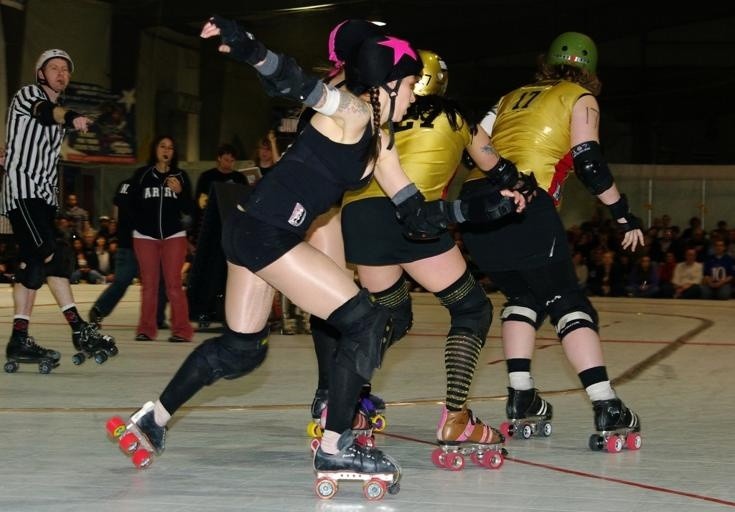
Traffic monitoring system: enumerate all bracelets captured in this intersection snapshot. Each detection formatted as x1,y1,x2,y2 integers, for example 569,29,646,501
62,110,84,130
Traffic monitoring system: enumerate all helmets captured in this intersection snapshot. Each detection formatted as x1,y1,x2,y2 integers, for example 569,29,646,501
412,49,449,97
344,28,423,95
546,32,598,76
35,49,75,82
328,19,376,66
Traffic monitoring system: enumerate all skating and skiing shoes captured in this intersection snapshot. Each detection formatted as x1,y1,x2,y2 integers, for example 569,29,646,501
5,331,61,373
106,401,168,470
72,321,119,365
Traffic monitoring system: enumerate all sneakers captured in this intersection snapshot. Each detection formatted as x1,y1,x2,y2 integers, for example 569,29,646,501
90,309,104,330
136,334,150,340
168,335,184,342
158,319,171,329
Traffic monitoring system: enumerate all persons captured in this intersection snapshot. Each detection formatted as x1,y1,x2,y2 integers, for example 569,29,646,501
0,120,733,341
3,48,118,374
289,20,395,434
457,28,652,432
97,11,529,483
308,45,540,449
